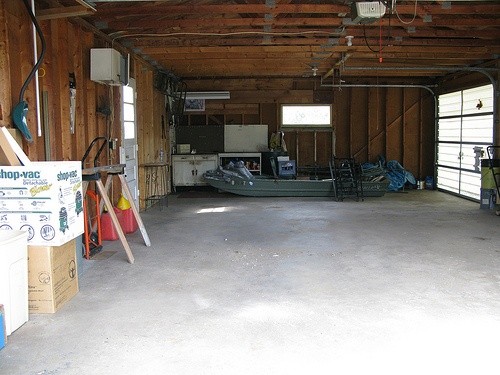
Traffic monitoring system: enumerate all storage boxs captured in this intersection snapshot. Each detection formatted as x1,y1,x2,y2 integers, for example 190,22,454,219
0,161,84,246
0,230,29,336
278,160,295,175
28,239,79,314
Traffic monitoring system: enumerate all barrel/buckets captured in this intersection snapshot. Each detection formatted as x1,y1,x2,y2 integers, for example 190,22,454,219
117,206,138,233
100,210,118,241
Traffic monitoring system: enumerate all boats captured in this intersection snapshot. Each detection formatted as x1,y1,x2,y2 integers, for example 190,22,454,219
202,160,392,198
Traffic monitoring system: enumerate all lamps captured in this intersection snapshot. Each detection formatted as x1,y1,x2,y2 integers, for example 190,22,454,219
175,91,231,100
345,35,354,46
311,67,318,76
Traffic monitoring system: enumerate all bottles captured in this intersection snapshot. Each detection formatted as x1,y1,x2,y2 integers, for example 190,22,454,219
417,181,419,189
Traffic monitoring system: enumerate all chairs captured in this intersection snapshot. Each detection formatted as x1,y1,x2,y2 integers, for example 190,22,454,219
329,153,364,201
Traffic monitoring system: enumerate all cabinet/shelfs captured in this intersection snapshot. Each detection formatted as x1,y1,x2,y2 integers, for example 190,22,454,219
146,163,168,210
218,153,261,175
172,155,218,186
